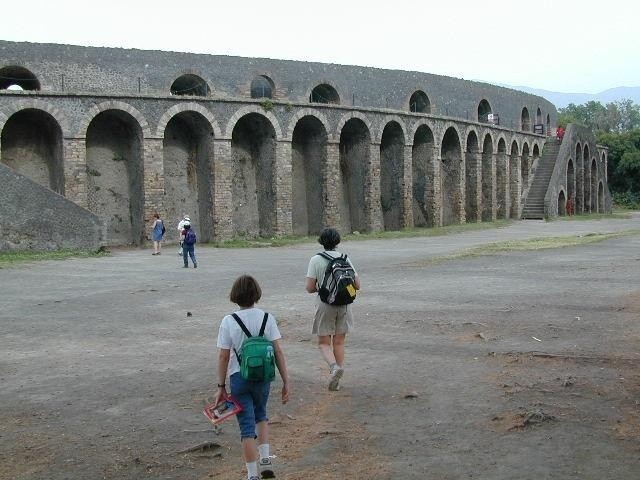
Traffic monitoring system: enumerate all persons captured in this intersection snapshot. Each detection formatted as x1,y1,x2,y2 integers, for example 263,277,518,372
213,273,291,480
178,217,191,255
556,125,564,146
181,224,198,267
178,214,189,229
151,213,164,256
305,228,361,392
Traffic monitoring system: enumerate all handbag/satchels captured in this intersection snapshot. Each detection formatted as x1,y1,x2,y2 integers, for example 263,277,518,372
162,225,166,236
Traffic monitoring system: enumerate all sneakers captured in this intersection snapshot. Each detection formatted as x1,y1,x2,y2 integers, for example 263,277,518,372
328,368,344,392
178,251,198,269
260,457,276,477
152,251,161,255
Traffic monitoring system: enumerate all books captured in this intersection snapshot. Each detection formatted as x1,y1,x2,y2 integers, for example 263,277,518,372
204,395,241,424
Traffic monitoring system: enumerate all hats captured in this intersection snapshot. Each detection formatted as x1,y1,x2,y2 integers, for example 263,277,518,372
184,215,191,226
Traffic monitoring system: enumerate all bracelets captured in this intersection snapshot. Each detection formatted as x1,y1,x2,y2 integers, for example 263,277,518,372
217,383,227,387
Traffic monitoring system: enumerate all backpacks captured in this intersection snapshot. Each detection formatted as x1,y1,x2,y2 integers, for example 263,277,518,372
313,250,356,306
185,229,196,245
231,313,276,380
